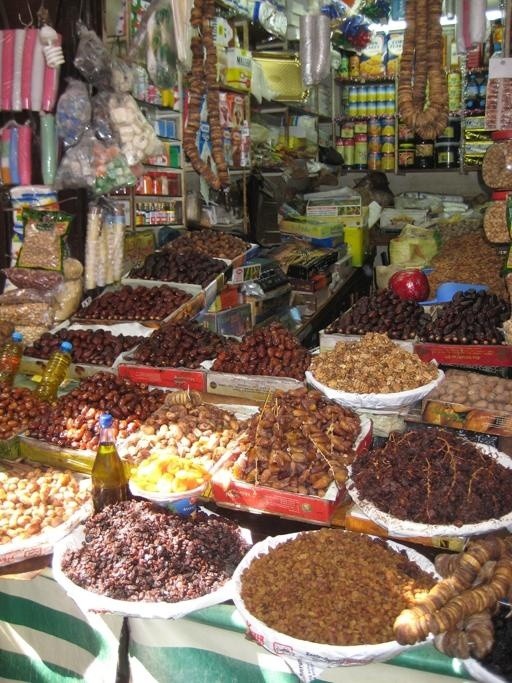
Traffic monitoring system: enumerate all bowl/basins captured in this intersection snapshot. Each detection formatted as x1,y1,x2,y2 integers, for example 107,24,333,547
127,475,206,524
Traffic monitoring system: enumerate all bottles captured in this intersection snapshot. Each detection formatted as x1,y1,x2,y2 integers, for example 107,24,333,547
89,415,131,514
135,201,178,226
0,331,22,388
493,18,506,52
35,339,73,405
103,203,126,225
484,190,511,245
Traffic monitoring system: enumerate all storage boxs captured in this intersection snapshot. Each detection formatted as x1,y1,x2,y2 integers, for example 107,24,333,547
2,192,511,436
2,374,512,667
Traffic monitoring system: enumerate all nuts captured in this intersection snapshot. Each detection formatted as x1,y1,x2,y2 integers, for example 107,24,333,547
0,228,512,683
482,140,512,189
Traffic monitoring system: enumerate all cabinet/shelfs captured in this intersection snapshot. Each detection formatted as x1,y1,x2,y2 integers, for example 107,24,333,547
391,63,467,178
332,70,400,178
250,23,333,182
182,7,254,236
104,0,190,234
